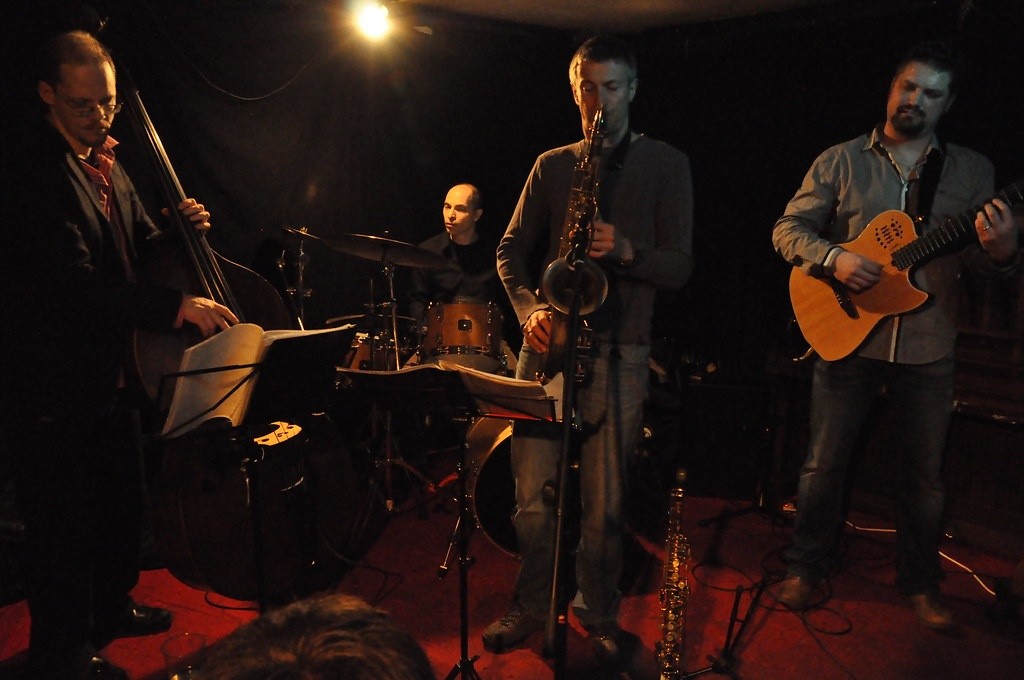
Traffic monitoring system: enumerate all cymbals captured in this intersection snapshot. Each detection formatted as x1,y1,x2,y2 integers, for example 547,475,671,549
269,223,323,246
320,232,451,268
325,313,417,331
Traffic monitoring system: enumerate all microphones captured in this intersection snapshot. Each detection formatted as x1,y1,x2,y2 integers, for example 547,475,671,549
565,248,577,271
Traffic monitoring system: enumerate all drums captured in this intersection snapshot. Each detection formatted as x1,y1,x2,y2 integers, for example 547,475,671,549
419,295,506,373
334,331,404,392
462,415,581,557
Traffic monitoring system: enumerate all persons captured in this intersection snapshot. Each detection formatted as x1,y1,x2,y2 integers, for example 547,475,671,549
481,33,703,666
0,32,240,680
771,46,1024,628
403,182,522,375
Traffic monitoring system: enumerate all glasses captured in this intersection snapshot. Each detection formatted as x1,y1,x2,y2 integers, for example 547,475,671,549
52,89,124,118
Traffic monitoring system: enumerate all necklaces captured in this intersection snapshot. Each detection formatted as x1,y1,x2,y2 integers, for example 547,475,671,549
899,161,915,171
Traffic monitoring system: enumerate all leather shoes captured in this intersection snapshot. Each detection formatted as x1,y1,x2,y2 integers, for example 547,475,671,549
96,596,174,636
29,655,128,680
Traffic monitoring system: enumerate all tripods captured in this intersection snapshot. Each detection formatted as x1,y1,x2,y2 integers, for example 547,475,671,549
681,573,770,680
340,305,561,680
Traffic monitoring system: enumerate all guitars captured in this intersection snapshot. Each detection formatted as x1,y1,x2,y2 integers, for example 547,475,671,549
788,180,1022,363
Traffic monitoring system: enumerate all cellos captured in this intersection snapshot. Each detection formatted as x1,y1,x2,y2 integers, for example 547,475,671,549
113,58,361,604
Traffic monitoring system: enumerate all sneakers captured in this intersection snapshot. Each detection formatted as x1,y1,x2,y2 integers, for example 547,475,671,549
481,596,546,652
587,620,625,665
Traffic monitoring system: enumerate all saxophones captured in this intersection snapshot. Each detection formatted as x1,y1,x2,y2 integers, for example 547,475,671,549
535,103,611,389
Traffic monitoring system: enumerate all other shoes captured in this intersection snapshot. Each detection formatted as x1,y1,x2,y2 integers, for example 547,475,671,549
777,574,817,605
910,591,952,628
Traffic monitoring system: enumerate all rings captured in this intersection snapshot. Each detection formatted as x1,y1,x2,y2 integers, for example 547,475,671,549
983,226,990,231
527,331,532,336
